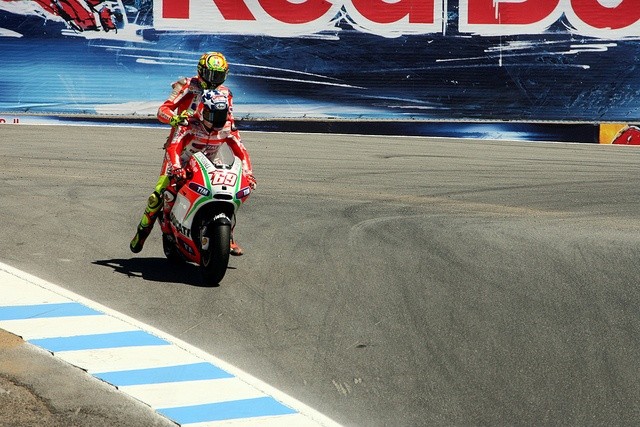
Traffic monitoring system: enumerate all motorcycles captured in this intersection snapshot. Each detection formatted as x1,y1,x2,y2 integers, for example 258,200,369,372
158,145,253,285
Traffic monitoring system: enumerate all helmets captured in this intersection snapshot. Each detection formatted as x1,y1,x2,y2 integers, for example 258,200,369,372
198,51,229,89
197,89,229,131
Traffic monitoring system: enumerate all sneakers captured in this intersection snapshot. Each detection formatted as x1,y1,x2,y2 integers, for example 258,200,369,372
130,229,151,253
229,237,243,256
160,215,171,234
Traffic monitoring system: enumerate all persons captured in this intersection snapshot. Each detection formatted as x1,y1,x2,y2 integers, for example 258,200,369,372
160,89,257,256
129,51,256,253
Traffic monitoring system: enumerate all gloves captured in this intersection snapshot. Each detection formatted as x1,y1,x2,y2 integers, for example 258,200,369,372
172,165,186,181
247,174,257,190
169,109,192,128
